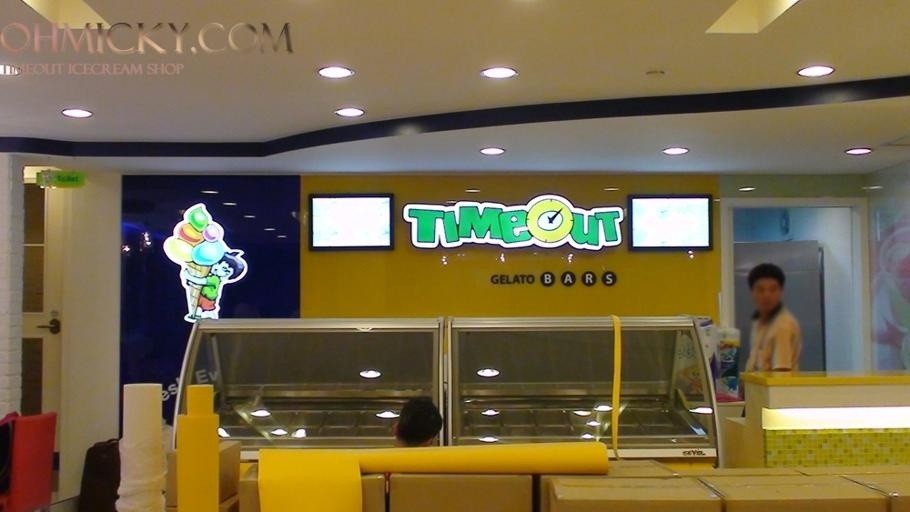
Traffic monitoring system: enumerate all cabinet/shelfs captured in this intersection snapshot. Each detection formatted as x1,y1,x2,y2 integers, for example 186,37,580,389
168,314,724,470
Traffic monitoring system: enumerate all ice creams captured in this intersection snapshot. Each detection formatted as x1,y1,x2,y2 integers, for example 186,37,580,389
164,203,225,319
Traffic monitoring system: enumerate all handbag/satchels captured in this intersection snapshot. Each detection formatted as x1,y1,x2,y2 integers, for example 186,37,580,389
80,439,120,512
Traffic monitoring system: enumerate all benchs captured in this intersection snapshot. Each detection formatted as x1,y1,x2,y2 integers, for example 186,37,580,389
239,440,618,512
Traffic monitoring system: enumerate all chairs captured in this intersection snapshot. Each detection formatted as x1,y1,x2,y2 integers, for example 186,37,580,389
0,411,55,512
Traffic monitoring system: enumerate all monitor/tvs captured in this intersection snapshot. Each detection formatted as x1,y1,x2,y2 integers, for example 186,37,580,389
628,193,711,251
307,192,395,251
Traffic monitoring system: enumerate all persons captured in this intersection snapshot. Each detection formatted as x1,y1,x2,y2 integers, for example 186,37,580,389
392,395,443,448
738,263,802,418
